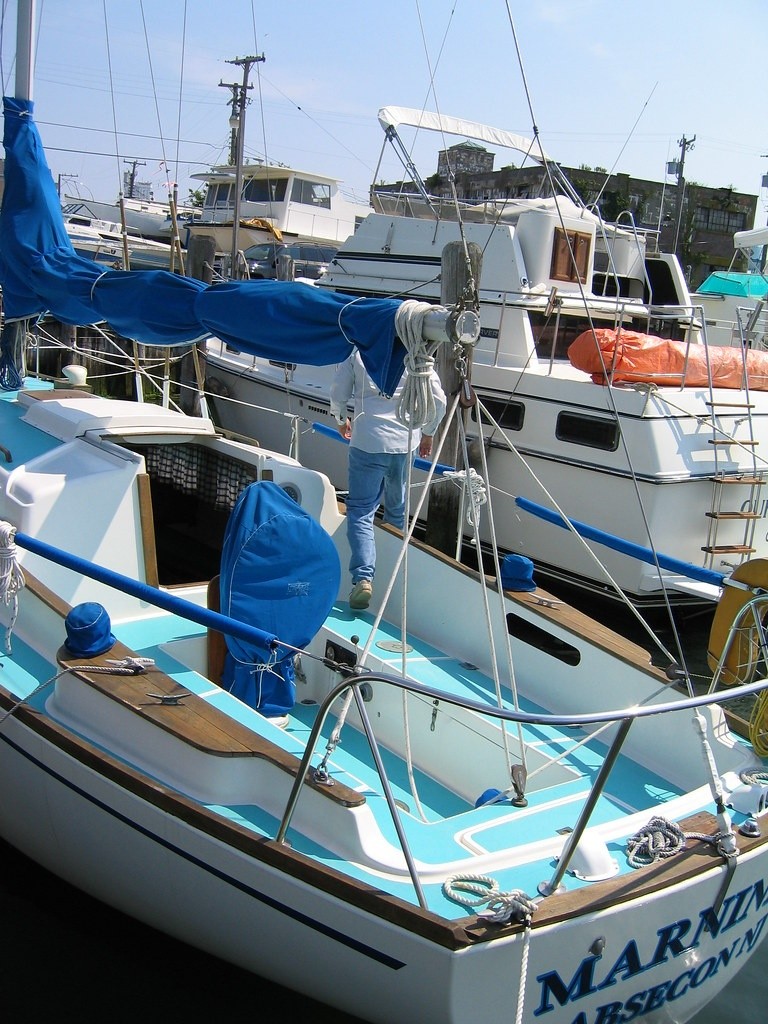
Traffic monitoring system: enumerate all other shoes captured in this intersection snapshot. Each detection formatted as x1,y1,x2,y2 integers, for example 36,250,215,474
349,580,372,609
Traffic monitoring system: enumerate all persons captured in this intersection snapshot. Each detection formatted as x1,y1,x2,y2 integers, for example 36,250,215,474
330,345,447,610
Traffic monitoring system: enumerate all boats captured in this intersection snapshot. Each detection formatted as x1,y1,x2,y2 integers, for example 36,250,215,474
0,0,768,1024
